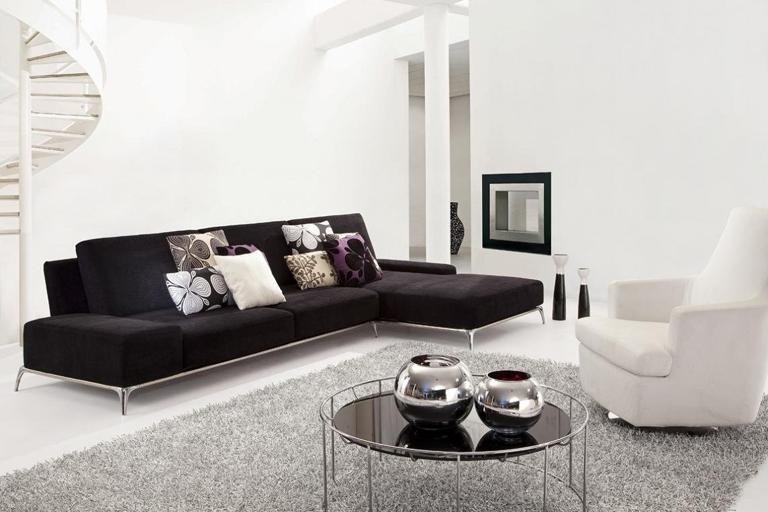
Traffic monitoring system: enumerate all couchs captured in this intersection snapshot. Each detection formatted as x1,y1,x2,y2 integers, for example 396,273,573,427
14,212,545,416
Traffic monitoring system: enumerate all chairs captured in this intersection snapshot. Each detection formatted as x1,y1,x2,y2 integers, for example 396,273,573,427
575,207,767,432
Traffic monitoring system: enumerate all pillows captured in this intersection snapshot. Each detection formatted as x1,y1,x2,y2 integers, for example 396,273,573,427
161,220,383,316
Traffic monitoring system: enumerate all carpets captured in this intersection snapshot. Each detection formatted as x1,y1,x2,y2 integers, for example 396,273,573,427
1,341,766,512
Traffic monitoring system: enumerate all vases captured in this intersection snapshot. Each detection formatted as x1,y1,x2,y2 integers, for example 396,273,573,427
551,253,591,321
450,202,463,254
